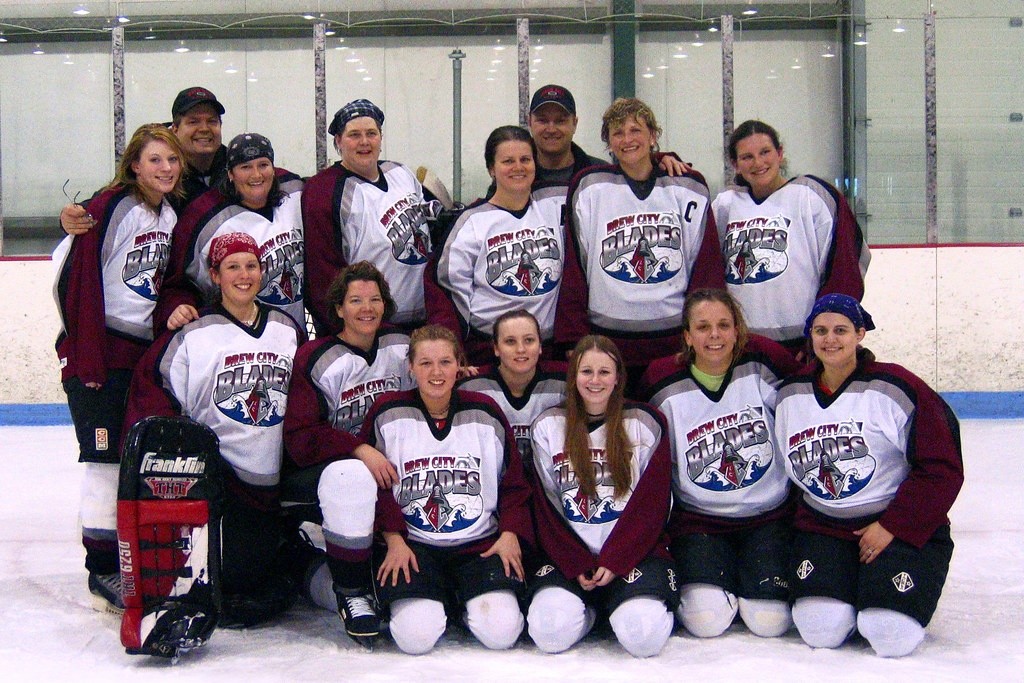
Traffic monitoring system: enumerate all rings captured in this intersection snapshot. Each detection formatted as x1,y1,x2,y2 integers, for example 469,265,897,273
868,549,872,554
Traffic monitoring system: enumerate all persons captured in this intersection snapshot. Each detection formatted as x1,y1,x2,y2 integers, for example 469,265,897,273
53,85,963,658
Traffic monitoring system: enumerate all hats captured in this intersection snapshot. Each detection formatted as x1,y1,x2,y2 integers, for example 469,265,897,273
172,87,225,118
530,84,576,114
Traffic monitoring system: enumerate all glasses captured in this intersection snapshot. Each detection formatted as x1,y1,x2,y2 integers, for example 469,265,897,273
62,178,98,227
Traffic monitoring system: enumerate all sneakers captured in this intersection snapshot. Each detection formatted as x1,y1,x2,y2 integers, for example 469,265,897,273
87,567,125,616
335,591,382,651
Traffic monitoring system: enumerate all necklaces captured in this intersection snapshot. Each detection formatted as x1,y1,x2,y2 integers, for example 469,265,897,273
587,412,604,417
241,303,257,327
428,406,448,416
